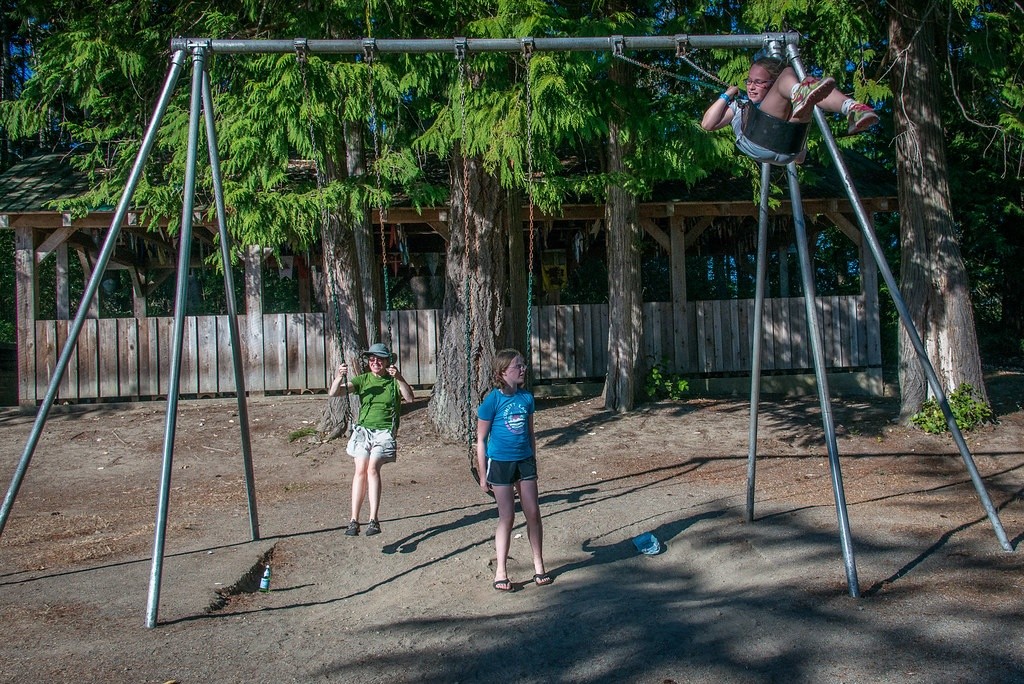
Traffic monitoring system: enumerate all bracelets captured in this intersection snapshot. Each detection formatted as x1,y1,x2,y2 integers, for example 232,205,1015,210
718,94,731,106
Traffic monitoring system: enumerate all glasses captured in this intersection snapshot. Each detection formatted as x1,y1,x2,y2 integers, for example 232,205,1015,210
509,365,528,369
742,78,773,87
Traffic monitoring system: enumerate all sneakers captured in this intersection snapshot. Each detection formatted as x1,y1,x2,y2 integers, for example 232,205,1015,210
345,518,360,537
847,103,879,134
792,76,835,118
366,520,381,536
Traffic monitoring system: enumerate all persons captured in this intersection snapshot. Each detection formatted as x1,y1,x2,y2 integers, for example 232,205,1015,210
329,343,414,537
701,55,877,166
476,349,553,592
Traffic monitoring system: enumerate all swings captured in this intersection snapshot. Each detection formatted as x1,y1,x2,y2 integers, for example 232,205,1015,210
614,53,814,158
293,47,399,464
455,48,535,500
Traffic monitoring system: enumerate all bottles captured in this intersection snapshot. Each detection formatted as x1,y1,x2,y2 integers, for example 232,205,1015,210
259,565,270,593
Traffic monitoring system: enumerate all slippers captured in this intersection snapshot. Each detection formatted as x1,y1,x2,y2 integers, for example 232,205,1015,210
533,574,552,585
493,578,513,592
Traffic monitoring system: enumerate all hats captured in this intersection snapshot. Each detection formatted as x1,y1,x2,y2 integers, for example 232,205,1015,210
362,343,397,364
